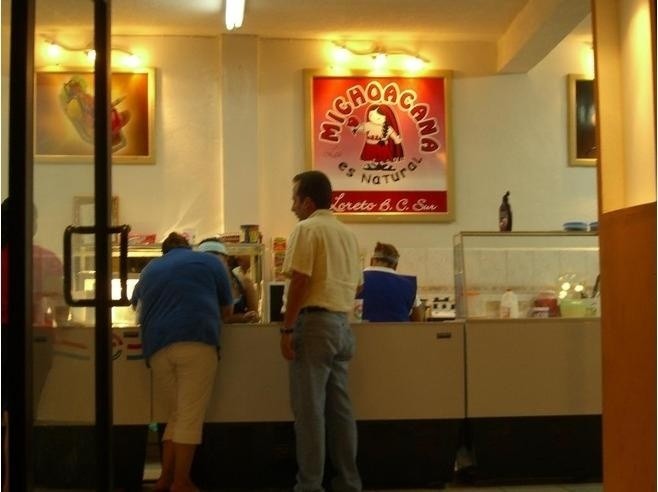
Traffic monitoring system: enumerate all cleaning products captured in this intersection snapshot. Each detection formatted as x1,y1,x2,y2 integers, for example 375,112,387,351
499,190,510,233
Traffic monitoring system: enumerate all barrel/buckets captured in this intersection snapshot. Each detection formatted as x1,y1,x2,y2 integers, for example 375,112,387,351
499,287,520,319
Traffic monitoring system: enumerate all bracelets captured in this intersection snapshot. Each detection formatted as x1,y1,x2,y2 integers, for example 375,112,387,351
280,327,294,334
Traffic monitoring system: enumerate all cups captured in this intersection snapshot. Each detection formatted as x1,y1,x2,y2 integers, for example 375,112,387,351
532,298,560,318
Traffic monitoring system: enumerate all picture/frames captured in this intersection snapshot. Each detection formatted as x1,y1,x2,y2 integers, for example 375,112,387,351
301,66,457,225
567,72,599,167
71,193,120,246
33,67,156,168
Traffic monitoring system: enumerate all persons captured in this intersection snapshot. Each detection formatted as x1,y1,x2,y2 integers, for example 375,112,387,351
131,229,235,492
278,169,366,492
2,196,72,492
196,236,261,324
355,241,425,322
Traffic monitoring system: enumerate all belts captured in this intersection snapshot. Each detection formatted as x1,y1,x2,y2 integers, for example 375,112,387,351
299,305,328,315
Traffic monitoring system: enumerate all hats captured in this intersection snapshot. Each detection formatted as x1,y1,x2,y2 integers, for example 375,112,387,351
197,241,226,256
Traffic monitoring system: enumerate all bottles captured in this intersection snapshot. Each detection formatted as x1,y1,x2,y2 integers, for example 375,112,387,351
497,189,514,232
496,286,522,319
